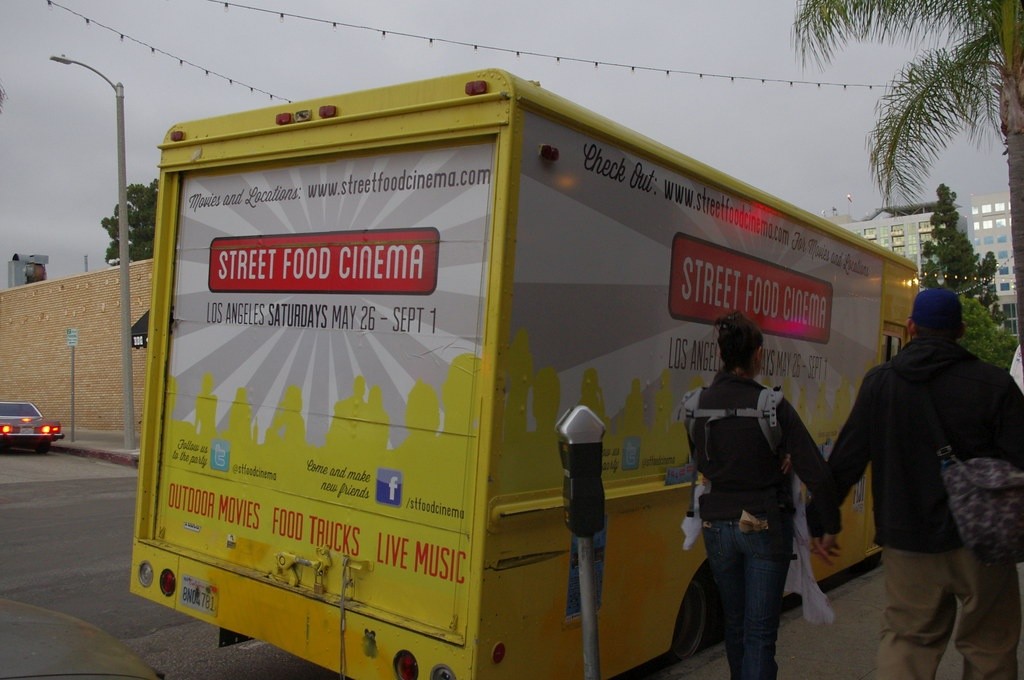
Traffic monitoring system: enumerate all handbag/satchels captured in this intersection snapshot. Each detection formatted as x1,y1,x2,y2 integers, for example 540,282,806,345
942,455,1024,564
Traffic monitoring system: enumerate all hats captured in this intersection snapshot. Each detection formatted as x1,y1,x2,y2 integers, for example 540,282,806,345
908,288,962,328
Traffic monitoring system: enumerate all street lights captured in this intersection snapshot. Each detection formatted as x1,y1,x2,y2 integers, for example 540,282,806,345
51,54,141,455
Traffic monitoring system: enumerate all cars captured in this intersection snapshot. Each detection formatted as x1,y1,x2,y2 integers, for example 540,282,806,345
1,400,66,456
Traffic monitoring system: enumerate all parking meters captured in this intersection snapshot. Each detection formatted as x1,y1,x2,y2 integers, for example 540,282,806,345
554,402,611,539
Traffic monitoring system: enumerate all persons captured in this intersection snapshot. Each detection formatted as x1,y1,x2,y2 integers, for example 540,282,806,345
806,288,1024,680
679,313,841,680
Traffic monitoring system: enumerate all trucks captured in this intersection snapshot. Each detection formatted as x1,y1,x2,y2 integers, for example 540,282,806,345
126,65,924,680
